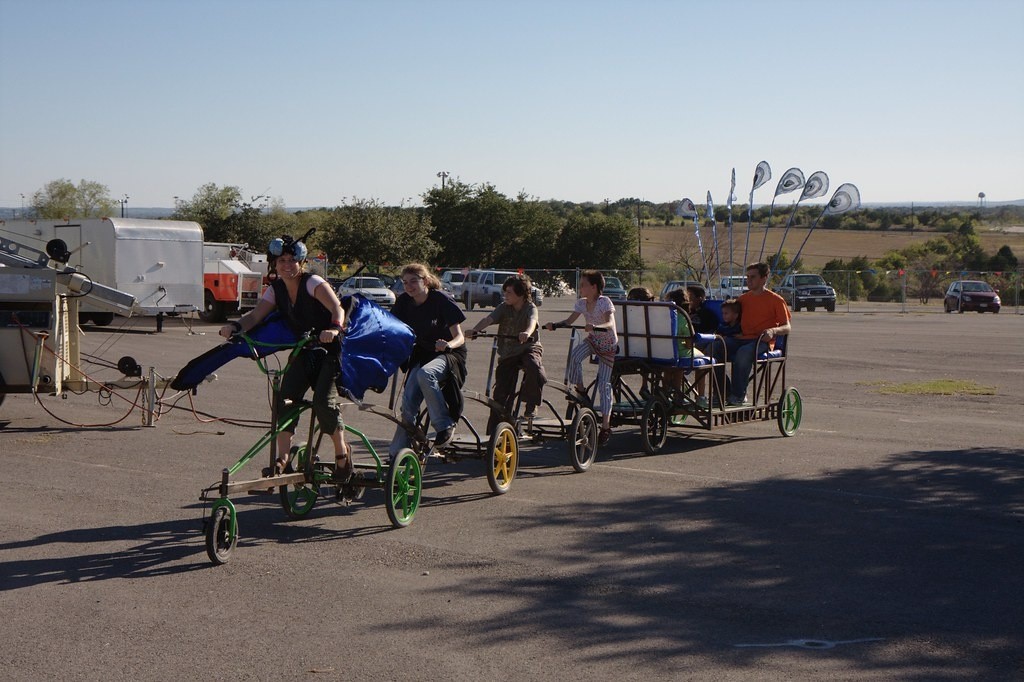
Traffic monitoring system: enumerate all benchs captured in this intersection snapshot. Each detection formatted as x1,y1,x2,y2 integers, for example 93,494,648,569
612,300,726,367
702,295,788,361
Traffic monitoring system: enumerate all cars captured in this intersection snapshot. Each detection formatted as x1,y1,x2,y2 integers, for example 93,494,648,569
337,277,395,307
602,277,627,301
659,281,716,302
943,281,1001,313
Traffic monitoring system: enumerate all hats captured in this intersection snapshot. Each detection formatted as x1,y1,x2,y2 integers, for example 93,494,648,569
266,235,307,266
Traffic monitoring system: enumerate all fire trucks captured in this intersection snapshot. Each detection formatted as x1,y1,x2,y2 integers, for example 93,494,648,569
165,242,281,322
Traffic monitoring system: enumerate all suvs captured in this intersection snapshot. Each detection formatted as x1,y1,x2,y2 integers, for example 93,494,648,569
439,271,465,302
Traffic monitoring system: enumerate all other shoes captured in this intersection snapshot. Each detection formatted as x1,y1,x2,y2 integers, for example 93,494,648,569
696,396,707,407
433,422,456,448
712,394,747,405
524,403,537,418
598,426,611,446
334,443,351,480
262,457,287,477
565,386,586,402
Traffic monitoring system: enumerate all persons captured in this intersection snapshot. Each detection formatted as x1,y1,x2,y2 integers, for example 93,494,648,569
716,263,792,405
464,276,546,447
627,287,653,301
378,263,467,473
664,285,720,409
546,270,618,444
220,235,352,486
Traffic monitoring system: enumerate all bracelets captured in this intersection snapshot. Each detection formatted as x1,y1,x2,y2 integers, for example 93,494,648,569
231,321,242,332
771,328,774,334
330,320,343,332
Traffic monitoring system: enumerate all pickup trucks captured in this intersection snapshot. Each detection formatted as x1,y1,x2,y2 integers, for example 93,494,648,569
460,271,543,309
332,272,396,292
705,276,750,299
772,274,836,312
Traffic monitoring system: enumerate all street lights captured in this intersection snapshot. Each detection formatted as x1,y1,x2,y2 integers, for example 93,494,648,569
118,200,128,218
437,172,449,188
